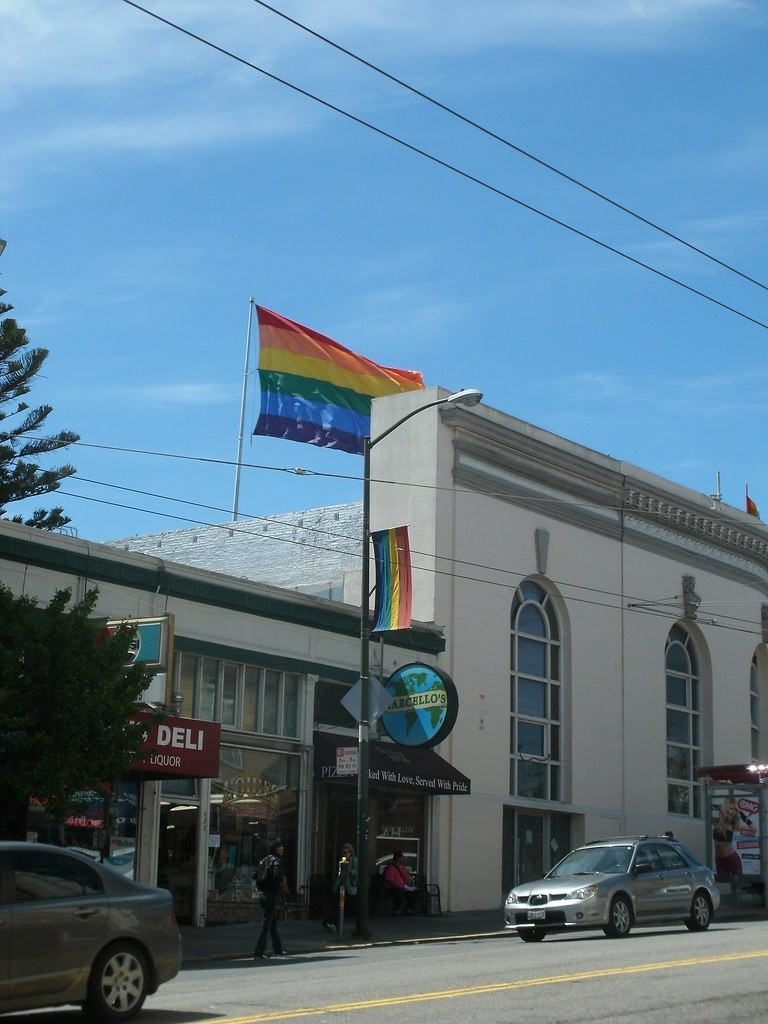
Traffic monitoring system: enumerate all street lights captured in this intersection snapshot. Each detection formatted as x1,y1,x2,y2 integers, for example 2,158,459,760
357,389,485,945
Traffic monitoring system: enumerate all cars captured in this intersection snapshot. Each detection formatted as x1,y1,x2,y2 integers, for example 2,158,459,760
374,852,419,875
505,836,720,942
0,841,183,1022
69,838,141,882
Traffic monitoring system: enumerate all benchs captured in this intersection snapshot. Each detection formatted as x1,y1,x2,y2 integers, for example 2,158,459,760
373,872,442,916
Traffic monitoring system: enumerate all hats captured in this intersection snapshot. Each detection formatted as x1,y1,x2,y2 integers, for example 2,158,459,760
270,841,283,853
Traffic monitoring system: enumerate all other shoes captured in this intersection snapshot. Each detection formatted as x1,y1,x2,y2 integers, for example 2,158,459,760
273,950,287,956
254,955,267,961
326,923,336,932
407,910,414,916
395,911,400,917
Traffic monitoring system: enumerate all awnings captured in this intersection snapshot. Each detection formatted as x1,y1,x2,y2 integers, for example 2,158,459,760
313,731,471,794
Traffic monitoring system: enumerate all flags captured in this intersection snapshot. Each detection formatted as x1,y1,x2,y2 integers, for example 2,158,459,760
371,526,412,632
254,304,426,456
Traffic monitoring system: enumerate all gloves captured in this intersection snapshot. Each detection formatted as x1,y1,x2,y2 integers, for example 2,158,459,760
739,811,751,826
711,804,721,810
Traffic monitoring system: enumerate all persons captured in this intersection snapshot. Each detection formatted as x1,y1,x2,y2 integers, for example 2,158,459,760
326,844,359,933
252,843,288,958
383,853,422,915
711,796,758,875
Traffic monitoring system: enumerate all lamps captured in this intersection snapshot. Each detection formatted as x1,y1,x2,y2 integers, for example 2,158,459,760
221,766,282,819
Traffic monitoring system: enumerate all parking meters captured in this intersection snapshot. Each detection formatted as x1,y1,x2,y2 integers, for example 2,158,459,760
337,860,349,937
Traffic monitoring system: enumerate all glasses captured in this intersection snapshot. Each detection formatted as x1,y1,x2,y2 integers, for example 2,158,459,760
341,851,347,855
